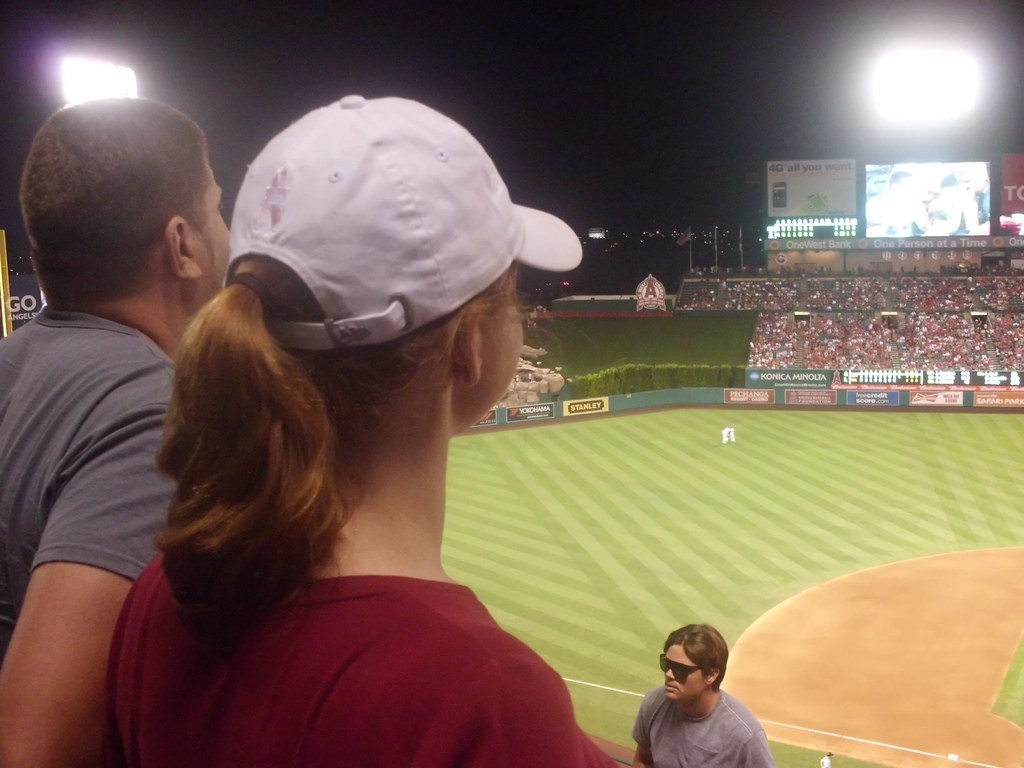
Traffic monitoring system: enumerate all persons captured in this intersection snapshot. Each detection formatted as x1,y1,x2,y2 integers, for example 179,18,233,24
677,264,1024,372
866,170,978,237
105,94,620,768
722,426,735,444
0,98,231,768
632,624,776,768
820,752,834,768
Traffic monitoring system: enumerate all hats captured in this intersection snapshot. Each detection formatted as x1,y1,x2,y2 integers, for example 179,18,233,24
222,94,583,353
826,752,833,756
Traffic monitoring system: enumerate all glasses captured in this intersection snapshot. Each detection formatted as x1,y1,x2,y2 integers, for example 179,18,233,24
659,653,703,685
